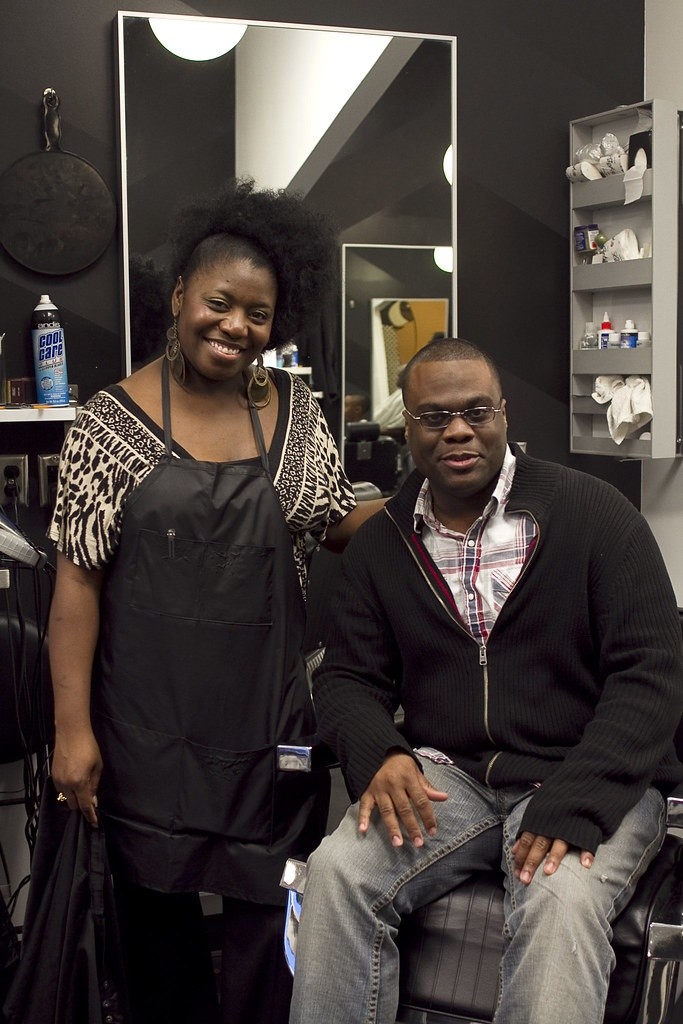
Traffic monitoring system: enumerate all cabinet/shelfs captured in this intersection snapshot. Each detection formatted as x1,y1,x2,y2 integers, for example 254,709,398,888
568,99,679,458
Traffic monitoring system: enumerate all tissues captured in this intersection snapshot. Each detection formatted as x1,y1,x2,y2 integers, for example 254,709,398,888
622,130,653,206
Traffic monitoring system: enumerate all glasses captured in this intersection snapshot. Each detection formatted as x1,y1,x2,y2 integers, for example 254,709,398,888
405,406,502,430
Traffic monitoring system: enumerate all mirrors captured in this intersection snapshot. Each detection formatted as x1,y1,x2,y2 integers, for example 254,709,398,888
338,244,450,479
117,10,460,481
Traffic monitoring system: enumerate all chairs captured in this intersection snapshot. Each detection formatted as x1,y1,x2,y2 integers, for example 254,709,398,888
375,607,683,1024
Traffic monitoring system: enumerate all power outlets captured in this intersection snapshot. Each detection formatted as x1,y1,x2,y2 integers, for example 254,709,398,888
0,455,29,508
40,453,67,513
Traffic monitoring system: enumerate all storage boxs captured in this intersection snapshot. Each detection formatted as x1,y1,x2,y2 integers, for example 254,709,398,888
6,377,36,405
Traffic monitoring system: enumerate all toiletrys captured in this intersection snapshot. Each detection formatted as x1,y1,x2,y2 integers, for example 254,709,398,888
578,311,652,351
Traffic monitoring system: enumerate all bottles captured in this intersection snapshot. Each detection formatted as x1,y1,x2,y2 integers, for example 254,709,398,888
32,295,69,407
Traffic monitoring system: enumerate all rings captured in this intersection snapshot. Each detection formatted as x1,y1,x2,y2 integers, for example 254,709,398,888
57,792,69,802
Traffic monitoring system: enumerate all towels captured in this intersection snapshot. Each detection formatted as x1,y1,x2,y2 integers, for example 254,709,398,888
590,374,654,446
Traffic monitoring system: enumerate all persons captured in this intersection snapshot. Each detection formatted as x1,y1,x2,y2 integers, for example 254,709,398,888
45,180,393,1024
289,339,683,1024
375,365,405,428
345,392,370,423
